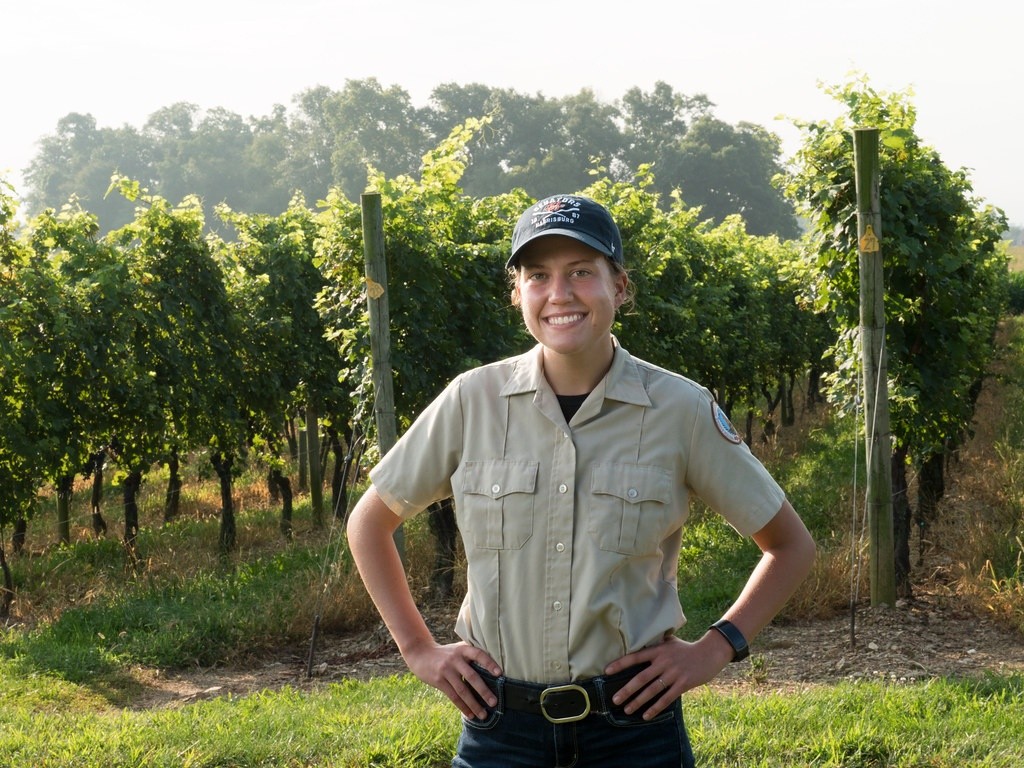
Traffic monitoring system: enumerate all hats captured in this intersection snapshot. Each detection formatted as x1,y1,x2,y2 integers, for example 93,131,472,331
504,194,624,272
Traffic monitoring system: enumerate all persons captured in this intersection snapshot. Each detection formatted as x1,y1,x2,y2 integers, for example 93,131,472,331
347,194,817,768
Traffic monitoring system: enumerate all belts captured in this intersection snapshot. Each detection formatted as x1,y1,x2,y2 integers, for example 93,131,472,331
464,675,630,724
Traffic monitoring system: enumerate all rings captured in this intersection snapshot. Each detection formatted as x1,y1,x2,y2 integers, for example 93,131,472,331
656,678,667,689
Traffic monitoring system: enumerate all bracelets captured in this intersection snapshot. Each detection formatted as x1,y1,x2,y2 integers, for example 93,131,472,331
708,620,750,662
